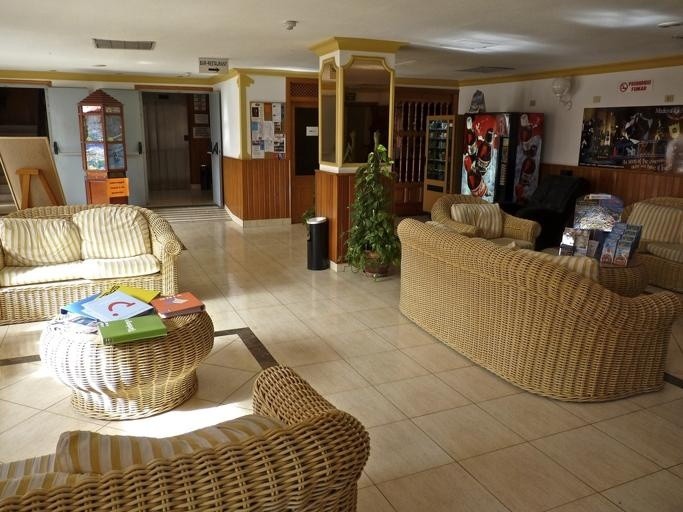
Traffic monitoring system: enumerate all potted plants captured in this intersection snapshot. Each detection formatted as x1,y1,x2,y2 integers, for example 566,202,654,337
342,143,402,278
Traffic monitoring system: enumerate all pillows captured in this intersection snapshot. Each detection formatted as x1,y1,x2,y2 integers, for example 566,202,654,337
451,203,503,240
516,249,600,285
625,203,682,244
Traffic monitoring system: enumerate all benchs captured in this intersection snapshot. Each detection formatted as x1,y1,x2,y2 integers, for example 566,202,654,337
0,364,372,512
0,205,183,324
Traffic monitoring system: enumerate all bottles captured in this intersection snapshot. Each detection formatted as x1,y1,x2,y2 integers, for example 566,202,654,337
520,113,537,155
464,156,488,196
466,116,478,161
516,144,537,185
476,127,494,175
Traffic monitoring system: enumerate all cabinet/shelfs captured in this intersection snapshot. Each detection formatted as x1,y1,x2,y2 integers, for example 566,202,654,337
421,113,464,213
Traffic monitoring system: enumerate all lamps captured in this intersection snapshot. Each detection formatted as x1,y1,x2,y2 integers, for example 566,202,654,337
551,77,573,111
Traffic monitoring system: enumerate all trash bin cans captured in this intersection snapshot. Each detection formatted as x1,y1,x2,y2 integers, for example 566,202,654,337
307,216,329,270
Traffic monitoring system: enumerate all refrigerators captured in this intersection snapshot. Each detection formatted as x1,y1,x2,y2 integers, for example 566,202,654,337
459,110,545,211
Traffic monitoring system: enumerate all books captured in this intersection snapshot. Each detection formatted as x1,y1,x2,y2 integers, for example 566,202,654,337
60,285,205,347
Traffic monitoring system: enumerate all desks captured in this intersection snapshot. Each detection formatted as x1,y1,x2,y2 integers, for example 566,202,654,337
542,245,649,298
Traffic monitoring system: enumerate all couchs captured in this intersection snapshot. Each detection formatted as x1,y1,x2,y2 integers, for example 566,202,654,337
619,197,682,294
397,218,683,401
431,194,541,253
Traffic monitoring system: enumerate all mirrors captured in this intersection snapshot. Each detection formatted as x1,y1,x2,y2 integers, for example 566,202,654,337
316,54,395,169
